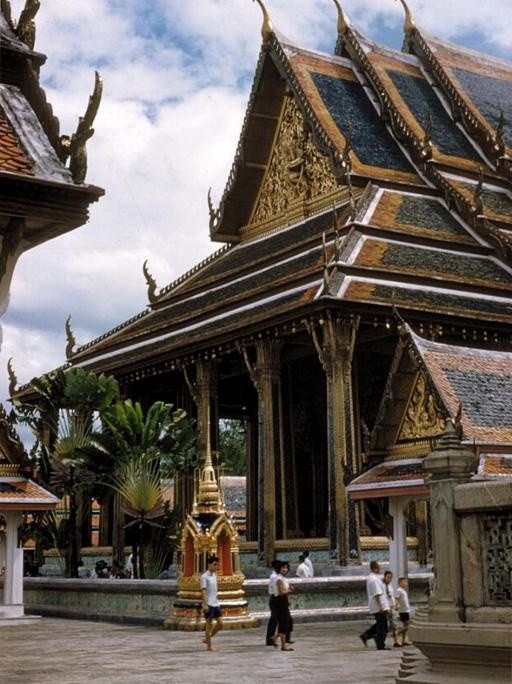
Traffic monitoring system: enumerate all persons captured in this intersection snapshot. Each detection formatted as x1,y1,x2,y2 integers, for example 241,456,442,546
359,560,392,650
199,555,224,652
265,560,295,646
303,549,314,577
270,559,295,651
296,554,310,576
78,552,140,578
392,575,413,645
379,570,403,647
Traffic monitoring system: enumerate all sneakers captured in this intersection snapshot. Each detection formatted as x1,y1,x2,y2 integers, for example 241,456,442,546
267,637,294,651
202,639,216,651
394,641,412,647
360,636,390,650
